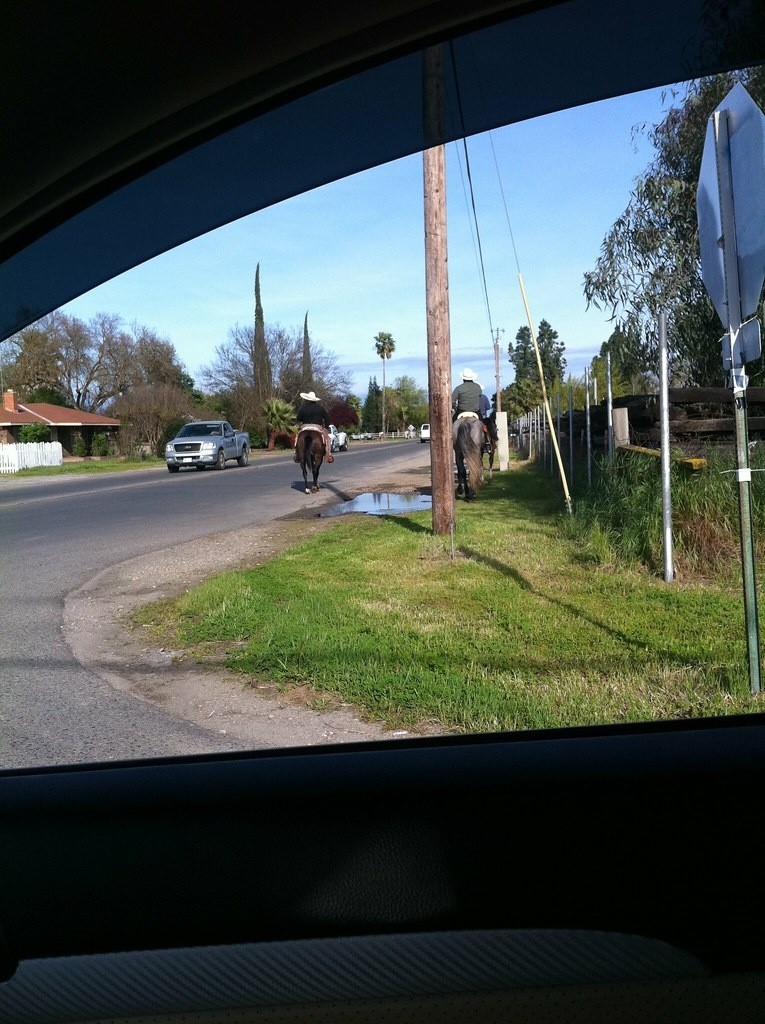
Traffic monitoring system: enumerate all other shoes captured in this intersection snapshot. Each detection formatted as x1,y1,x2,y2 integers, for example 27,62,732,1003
486,444,491,452
295,453,300,462
325,456,334,463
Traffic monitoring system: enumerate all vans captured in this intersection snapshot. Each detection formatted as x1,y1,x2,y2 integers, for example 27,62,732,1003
420,424,430,443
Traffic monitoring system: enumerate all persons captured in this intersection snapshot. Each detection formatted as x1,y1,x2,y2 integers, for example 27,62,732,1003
293,391,334,464
478,385,490,419
450,367,492,454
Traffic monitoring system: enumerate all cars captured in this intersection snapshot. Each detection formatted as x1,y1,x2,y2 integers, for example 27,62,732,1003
351,433,360,440
361,433,373,440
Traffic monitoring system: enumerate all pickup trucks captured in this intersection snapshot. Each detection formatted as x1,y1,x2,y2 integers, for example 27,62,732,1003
328,425,348,453
165,421,250,473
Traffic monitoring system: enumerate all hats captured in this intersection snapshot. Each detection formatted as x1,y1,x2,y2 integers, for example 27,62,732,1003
460,368,478,380
475,382,485,390
300,392,321,401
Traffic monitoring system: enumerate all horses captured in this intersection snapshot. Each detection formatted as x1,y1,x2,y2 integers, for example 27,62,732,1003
295,425,326,495
452,414,499,503
